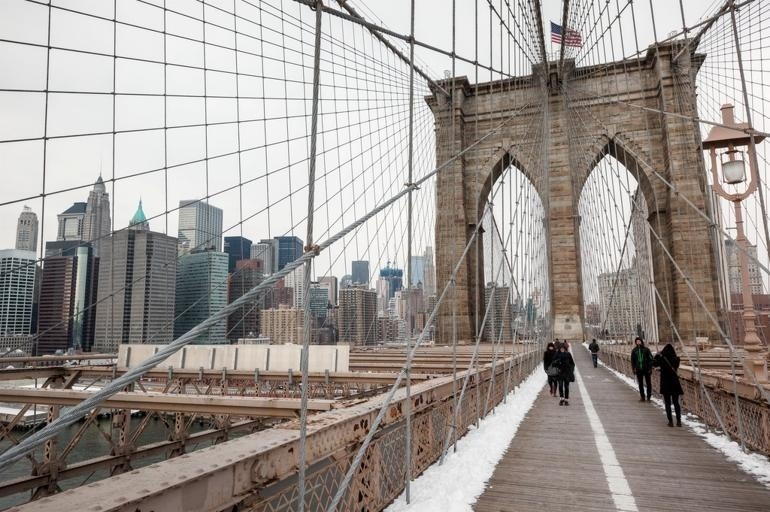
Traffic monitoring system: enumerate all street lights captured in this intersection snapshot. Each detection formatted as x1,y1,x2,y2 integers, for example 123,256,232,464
697,104,770,384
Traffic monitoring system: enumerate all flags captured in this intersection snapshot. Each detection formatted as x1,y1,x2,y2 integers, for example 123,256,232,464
551,23,582,47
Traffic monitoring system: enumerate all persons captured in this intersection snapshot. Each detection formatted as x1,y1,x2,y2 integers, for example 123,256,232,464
544,342,557,397
563,339,569,352
589,339,599,368
552,343,575,406
652,344,684,427
631,338,654,403
553,338,560,352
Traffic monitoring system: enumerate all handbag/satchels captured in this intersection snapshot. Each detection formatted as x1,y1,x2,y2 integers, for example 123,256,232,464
547,366,559,375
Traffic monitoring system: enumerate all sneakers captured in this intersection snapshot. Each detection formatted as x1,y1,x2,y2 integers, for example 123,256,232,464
565,401,568,405
559,400,564,405
